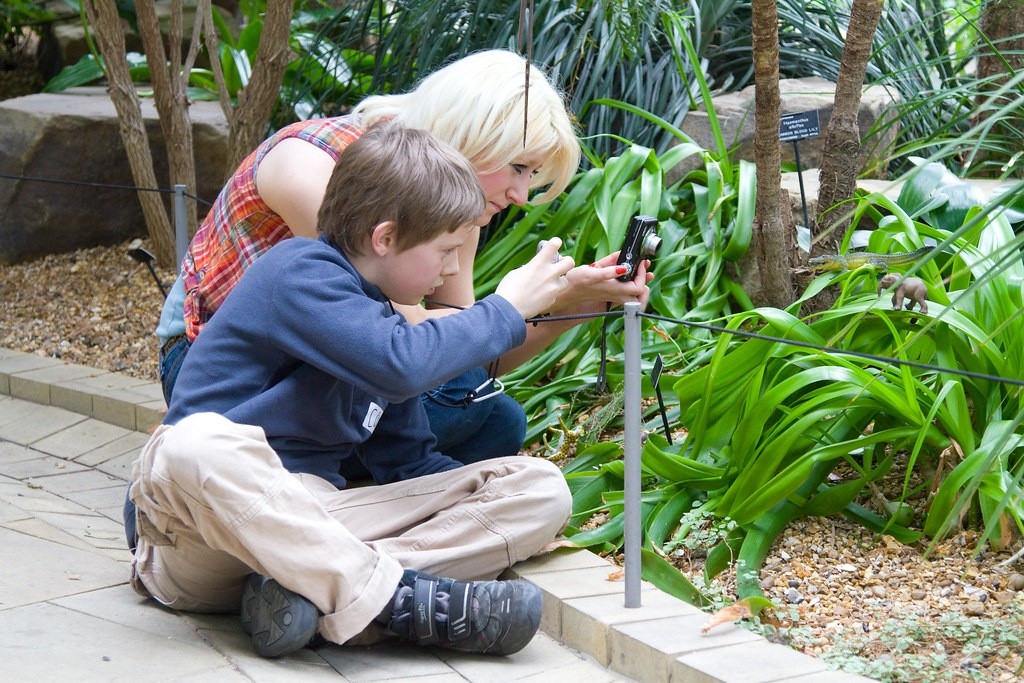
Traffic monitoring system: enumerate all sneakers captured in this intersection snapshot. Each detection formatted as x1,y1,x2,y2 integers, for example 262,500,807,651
241,572,322,657
374,569,542,654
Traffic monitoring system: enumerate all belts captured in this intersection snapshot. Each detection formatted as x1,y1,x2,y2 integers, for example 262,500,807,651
164,334,186,355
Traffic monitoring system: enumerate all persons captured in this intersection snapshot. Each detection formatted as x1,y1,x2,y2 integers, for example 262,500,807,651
152,48,656,408
122,123,574,659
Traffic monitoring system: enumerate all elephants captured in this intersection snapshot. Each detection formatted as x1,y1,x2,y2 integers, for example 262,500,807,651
877,273,928,314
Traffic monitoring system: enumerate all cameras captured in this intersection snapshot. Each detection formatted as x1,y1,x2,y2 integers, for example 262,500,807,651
537,240,562,264
617,216,663,282
